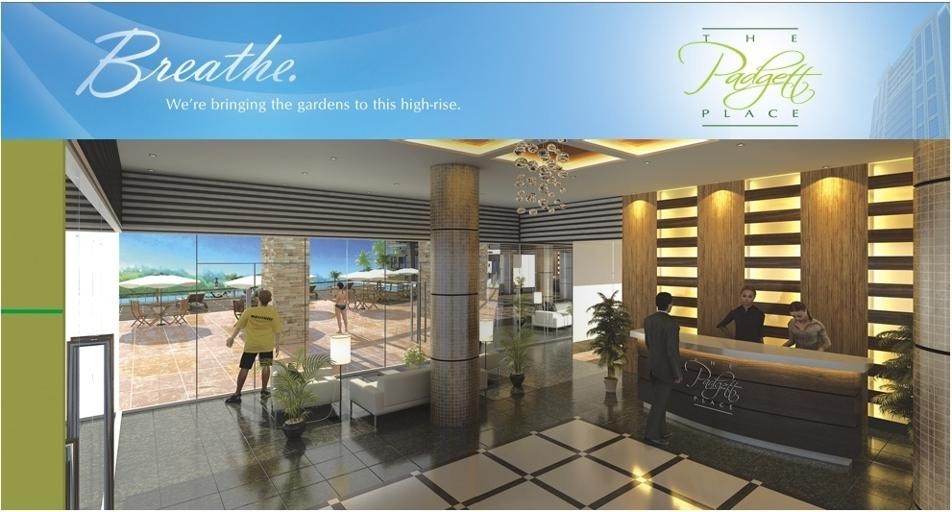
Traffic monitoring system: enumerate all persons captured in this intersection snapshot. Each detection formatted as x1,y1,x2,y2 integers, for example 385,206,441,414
334,282,348,334
643,292,688,446
716,285,765,344
225,290,282,404
782,301,833,352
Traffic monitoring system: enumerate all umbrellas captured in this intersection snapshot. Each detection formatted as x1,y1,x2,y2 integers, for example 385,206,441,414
119,267,419,289
